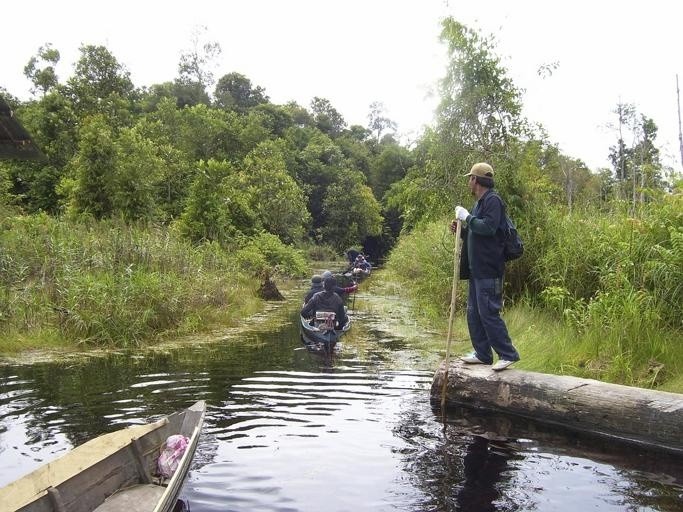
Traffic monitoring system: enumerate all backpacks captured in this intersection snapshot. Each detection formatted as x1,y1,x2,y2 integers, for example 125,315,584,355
485,194,524,262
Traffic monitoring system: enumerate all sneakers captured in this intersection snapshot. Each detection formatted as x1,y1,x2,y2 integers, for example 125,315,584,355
492,358,521,371
460,354,486,364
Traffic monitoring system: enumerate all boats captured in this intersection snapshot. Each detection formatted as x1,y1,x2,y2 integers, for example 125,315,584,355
332,273,358,294
300,301,350,352
346,256,371,280
1,400,206,512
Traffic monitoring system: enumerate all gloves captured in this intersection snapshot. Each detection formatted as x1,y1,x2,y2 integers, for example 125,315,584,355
455,206,470,221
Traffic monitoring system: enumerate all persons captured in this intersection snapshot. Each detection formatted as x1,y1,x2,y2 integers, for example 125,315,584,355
340,249,372,286
319,271,359,297
448,162,521,371
301,276,349,331
303,274,324,306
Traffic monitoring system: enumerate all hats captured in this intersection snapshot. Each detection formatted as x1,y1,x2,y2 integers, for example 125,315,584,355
464,162,494,179
311,271,333,287
